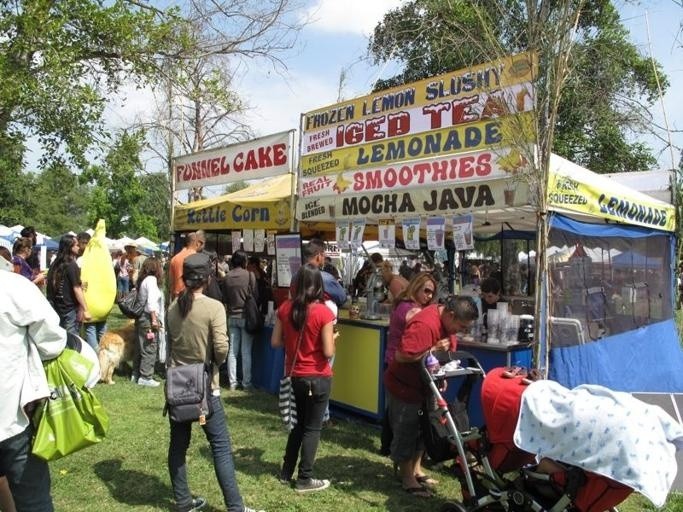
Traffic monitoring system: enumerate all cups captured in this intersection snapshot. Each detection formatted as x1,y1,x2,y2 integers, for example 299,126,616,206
487,302,520,343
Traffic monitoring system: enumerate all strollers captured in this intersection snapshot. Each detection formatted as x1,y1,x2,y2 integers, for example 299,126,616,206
418,349,633,512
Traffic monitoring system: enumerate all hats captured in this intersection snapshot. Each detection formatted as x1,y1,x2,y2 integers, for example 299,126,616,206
181,252,217,282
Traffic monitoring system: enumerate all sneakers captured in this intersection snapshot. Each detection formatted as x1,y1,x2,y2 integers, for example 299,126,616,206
278,470,298,483
136,377,162,388
292,475,333,494
174,496,203,510
130,374,138,382
236,505,267,512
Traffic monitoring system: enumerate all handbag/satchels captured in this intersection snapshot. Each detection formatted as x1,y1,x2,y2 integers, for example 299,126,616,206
115,291,148,320
30,348,109,463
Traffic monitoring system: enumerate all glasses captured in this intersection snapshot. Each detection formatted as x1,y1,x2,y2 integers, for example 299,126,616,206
420,286,438,298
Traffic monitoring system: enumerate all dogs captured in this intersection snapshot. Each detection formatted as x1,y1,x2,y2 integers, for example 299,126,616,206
98,321,140,385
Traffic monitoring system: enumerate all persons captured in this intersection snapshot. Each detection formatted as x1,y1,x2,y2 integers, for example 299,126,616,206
123,241,137,265
46,234,91,335
167,232,206,306
477,275,513,336
12,237,35,281
76,231,92,259
208,267,224,398
1,245,13,263
487,262,504,285
399,260,412,278
220,250,261,392
287,236,340,430
608,269,625,294
118,255,165,388
322,257,339,280
82,322,108,349
161,251,264,512
413,261,422,272
380,272,438,455
117,252,132,303
350,253,384,298
268,261,340,492
2,244,71,512
0,466,18,512
215,252,230,278
383,295,478,498
19,224,42,275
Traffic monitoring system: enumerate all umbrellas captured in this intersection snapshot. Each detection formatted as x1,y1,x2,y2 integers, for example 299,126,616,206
159,240,170,249
36,238,62,250
10,222,51,239
135,235,162,253
0,223,22,246
116,235,135,248
612,249,661,266
105,236,115,250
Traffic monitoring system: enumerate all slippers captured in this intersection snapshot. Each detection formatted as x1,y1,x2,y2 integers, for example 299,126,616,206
414,468,436,493
401,480,430,497
